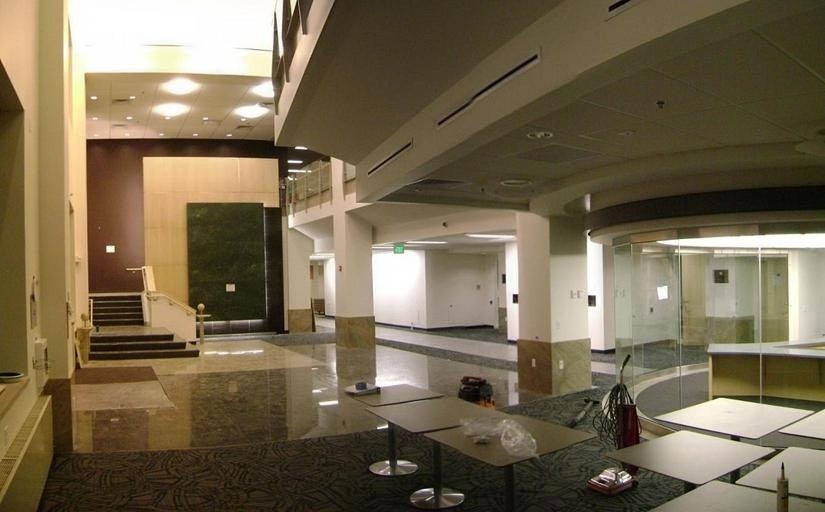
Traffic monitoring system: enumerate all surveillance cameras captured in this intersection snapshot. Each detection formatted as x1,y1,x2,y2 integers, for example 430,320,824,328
443,221,448,228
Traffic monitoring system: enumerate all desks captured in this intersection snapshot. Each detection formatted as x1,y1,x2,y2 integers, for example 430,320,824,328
776,408,824,441
656,395,814,484
735,446,825,500
344,384,446,477
365,396,507,512
424,414,598,512
604,428,776,495
645,478,824,512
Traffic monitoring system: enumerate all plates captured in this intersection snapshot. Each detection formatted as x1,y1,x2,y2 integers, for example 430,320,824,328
1,371,24,383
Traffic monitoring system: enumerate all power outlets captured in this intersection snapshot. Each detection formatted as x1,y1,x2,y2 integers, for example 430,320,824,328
559,360,564,370
532,359,536,368
